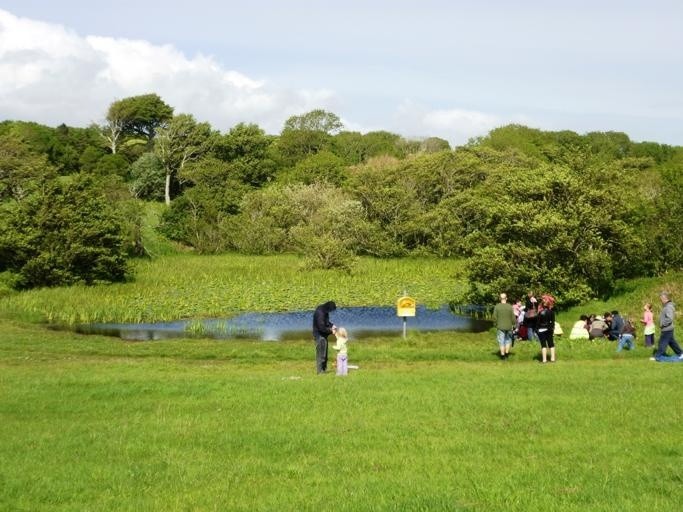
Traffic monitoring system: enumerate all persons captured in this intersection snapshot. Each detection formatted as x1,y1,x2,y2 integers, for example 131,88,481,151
313,301,336,375
569,311,637,351
649,292,683,362
640,302,655,349
492,290,563,363
332,327,348,376
